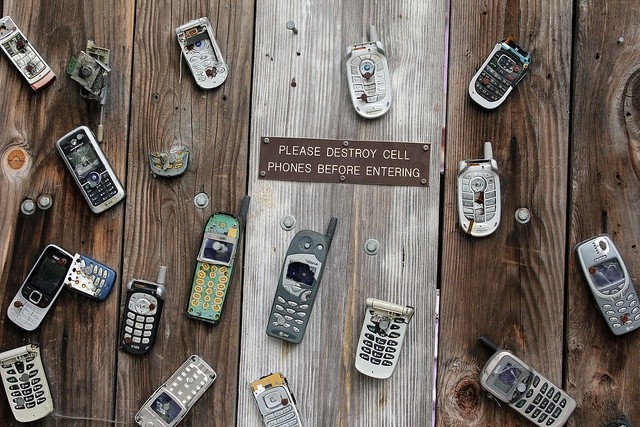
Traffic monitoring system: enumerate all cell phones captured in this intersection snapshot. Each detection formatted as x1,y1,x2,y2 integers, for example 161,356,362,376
266,218,337,343
345,26,391,119
120,266,166,354
469,36,531,110
175,16,227,90
55,125,126,214
0,16,55,91
250,374,303,427
355,298,414,380
0,342,54,423
185,196,250,326
457,142,500,238
7,244,76,332
135,355,217,427
479,335,576,427
574,233,637,336
